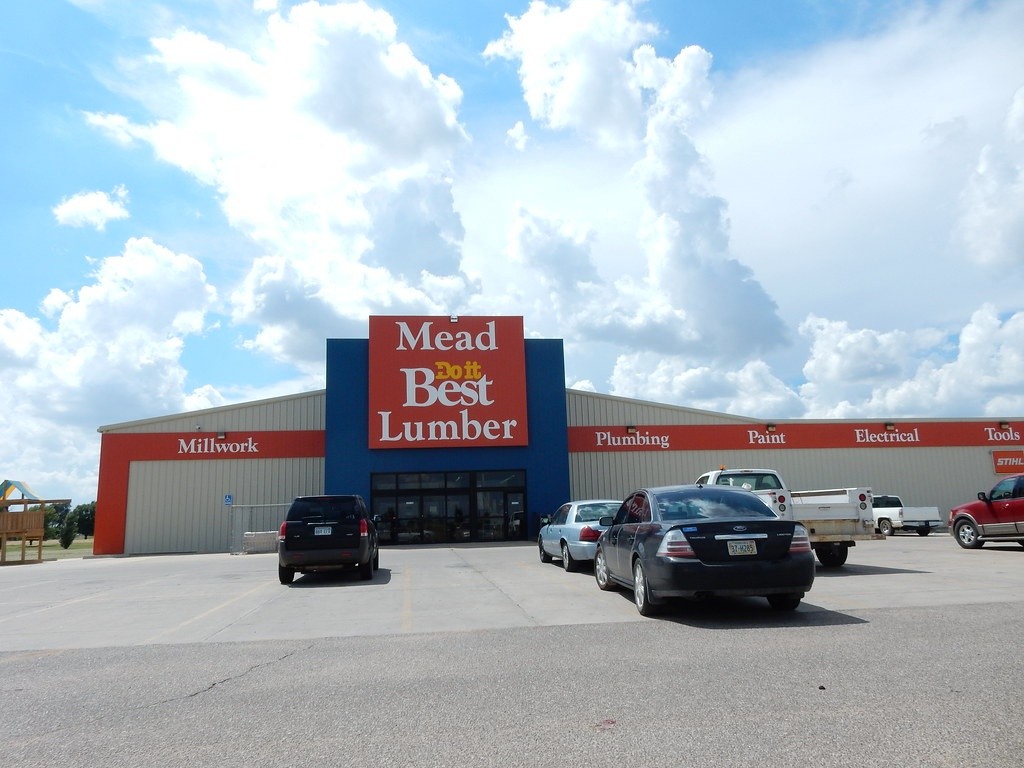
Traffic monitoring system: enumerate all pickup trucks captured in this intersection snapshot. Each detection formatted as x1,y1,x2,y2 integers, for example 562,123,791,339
871,495,944,537
692,469,886,569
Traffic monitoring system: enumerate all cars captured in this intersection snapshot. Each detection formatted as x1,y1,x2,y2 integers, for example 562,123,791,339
947,473,1024,550
451,524,471,540
593,485,816,618
538,499,623,572
508,511,524,536
376,524,437,544
477,524,498,539
277,495,382,584
